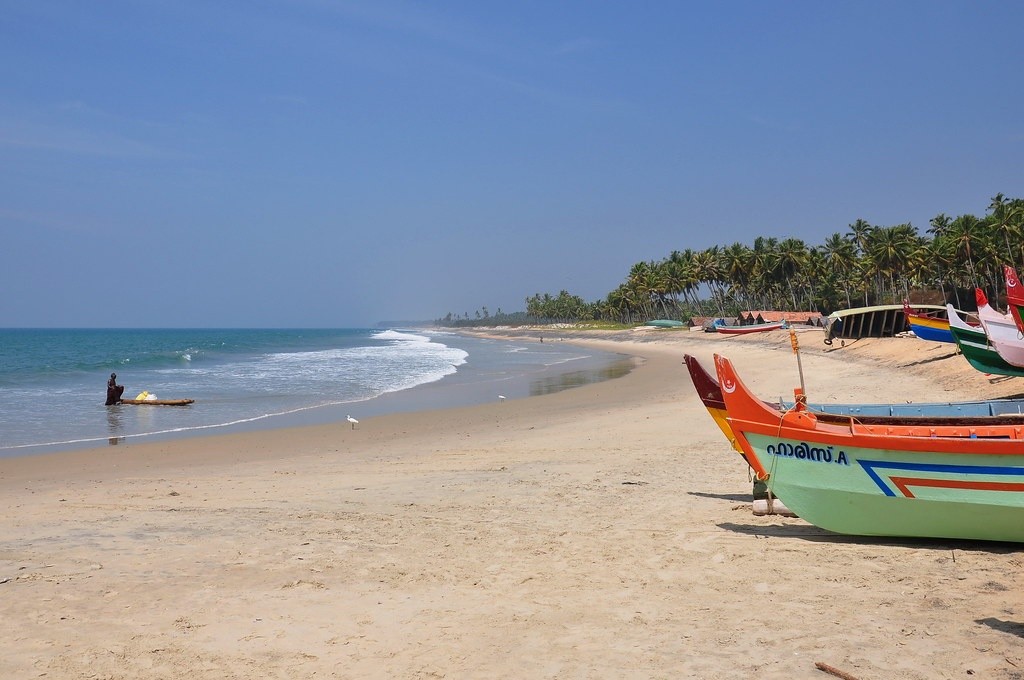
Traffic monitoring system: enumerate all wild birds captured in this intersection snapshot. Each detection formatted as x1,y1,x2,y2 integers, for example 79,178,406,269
497,395,506,402
346,415,359,430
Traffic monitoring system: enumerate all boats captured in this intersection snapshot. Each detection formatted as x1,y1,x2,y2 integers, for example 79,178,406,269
903,299,981,344
947,304,1024,378
975,289,1023,370
680,354,1023,499
824,305,979,346
715,318,786,334
711,353,1024,544
1003,264,1024,338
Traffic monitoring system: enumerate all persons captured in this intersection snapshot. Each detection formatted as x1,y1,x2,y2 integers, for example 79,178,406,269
106,373,117,404
